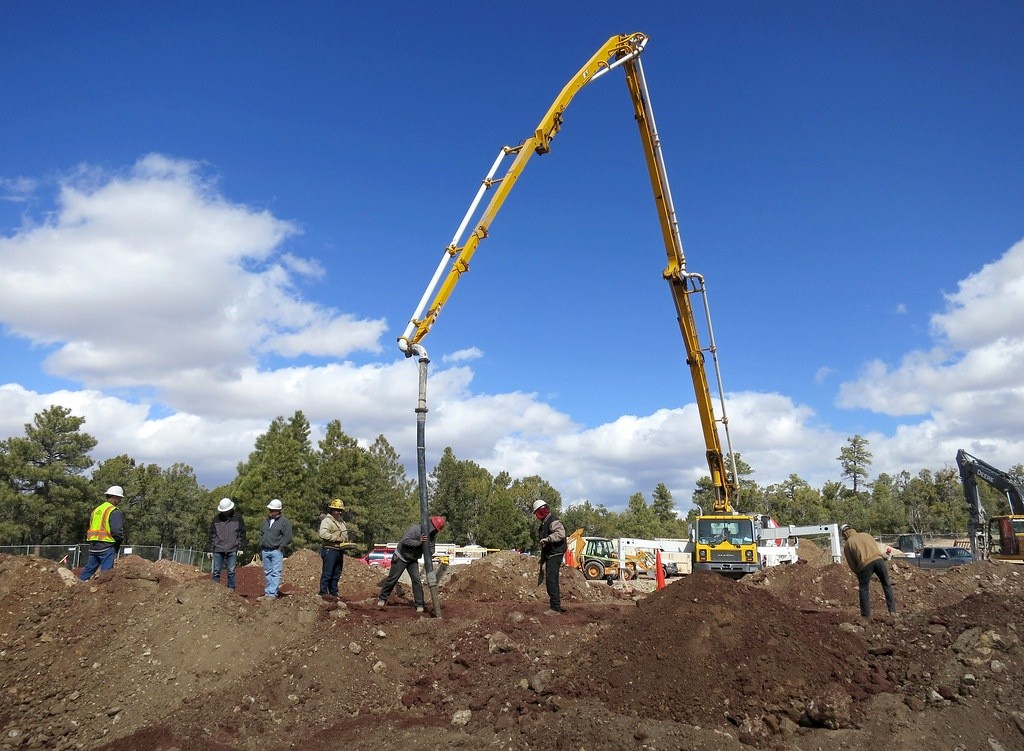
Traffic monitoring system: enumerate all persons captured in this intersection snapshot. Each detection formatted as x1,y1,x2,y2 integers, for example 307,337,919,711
319,499,348,598
840,524,896,618
80,486,123,581
531,500,568,615
378,516,446,612
207,498,246,589
511,547,521,554
256,499,292,604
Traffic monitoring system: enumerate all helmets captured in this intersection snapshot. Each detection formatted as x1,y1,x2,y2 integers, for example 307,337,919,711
328,499,345,509
104,485,124,497
217,497,234,511
839,524,852,537
266,499,282,509
886,548,891,553
532,500,548,514
431,516,446,532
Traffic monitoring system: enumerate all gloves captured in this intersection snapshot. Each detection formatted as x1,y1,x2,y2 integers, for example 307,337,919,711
237,550,243,556
207,552,214,558
539,539,548,546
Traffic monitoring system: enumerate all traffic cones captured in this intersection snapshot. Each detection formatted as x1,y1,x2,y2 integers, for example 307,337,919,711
657,551,666,591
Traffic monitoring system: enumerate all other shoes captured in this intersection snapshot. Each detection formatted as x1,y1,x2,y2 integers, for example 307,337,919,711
377,597,386,605
543,609,560,616
256,595,274,601
416,607,424,612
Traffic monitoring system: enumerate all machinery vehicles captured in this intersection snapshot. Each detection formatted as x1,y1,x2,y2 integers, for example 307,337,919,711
398,32,763,579
567,528,657,580
955,449,1024,565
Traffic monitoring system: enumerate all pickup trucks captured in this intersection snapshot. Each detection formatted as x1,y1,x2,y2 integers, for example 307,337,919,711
633,551,678,579
891,546,973,571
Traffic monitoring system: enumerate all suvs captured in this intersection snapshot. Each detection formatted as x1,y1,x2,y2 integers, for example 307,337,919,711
360,546,401,569
